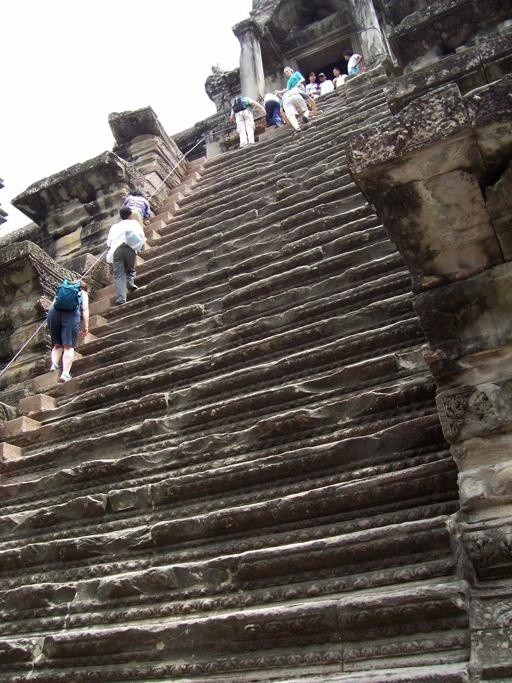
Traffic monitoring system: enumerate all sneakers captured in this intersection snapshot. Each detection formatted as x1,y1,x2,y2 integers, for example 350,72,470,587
127,284,138,292
50,362,56,370
57,374,72,384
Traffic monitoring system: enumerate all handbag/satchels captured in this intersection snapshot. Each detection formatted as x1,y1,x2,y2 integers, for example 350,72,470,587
124,227,143,253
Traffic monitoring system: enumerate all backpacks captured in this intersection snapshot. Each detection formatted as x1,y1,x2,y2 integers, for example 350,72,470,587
231,95,250,112
53,278,82,312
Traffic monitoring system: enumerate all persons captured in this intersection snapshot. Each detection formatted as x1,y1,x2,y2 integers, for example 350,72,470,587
230,95,266,148
264,49,362,136
122,191,150,255
107,207,146,304
47,280,89,381
